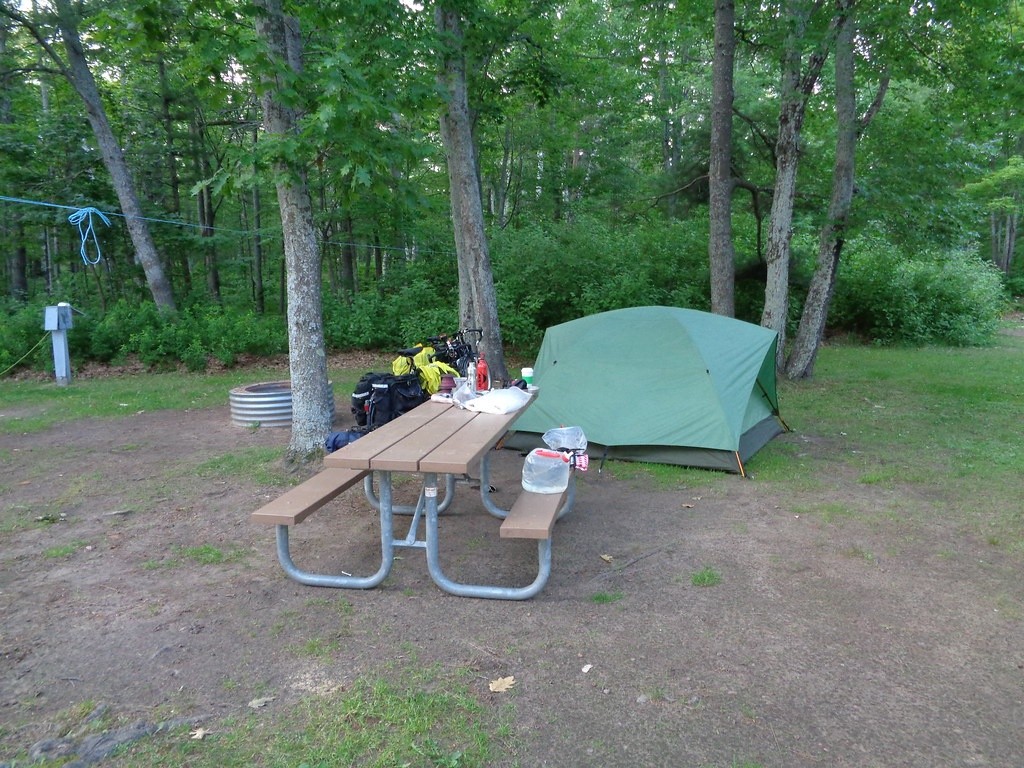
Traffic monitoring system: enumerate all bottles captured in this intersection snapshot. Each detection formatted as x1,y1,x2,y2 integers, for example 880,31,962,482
364,399,369,412
468,353,489,391
520,447,574,494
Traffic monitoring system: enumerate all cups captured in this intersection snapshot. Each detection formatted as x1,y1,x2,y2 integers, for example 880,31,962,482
521,367,533,389
454,377,467,391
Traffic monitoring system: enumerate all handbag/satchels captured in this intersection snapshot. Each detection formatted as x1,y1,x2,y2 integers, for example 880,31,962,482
370,374,427,426
351,372,395,426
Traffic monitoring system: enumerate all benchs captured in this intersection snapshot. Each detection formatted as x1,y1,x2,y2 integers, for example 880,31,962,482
251,466,576,601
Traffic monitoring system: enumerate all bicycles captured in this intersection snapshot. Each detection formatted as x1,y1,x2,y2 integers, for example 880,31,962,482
363,328,492,432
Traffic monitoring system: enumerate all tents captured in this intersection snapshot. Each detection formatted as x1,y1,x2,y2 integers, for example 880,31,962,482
497,306,792,478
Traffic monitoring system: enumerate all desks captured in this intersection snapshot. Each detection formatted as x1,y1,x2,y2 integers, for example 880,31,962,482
323,389,540,579
